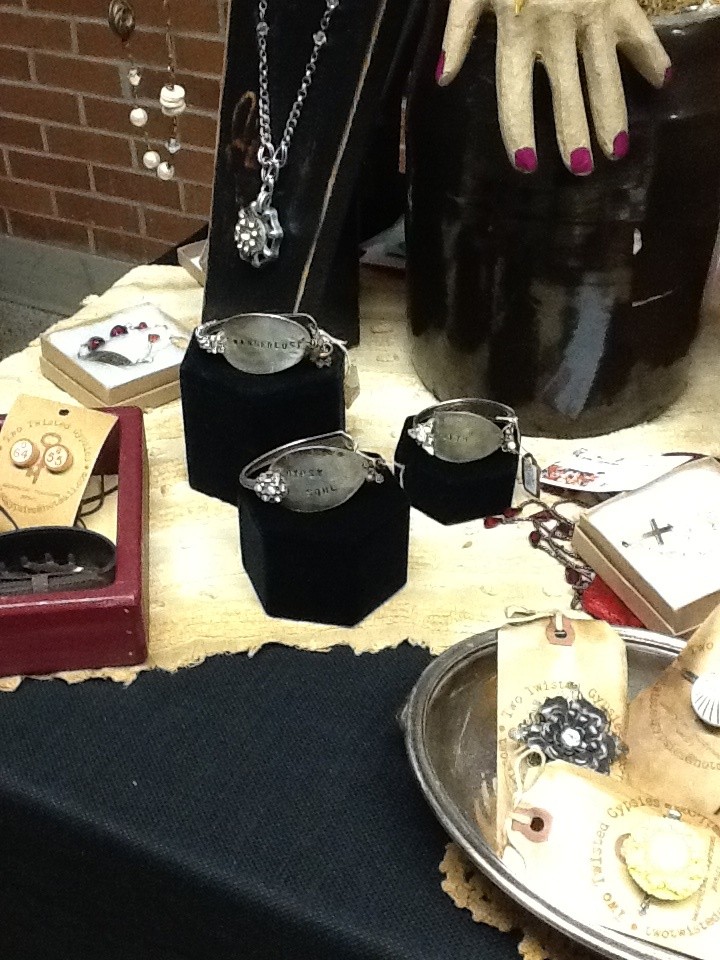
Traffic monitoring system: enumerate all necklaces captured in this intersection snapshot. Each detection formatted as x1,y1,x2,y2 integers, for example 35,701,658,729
486,500,595,610
235,0,346,266
77,322,188,366
109,0,185,181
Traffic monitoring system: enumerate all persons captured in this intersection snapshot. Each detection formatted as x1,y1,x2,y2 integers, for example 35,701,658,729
436,0,672,176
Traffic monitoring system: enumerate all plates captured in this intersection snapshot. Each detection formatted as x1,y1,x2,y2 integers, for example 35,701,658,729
406,619,690,960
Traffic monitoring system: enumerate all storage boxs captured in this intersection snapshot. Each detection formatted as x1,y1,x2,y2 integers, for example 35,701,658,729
570,453,720,637
39,303,195,412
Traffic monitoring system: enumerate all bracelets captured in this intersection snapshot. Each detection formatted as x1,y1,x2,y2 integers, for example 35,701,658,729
408,398,521,463
196,312,333,373
239,430,383,512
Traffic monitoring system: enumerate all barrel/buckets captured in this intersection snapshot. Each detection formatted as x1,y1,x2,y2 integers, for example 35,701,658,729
401,0,720,434
